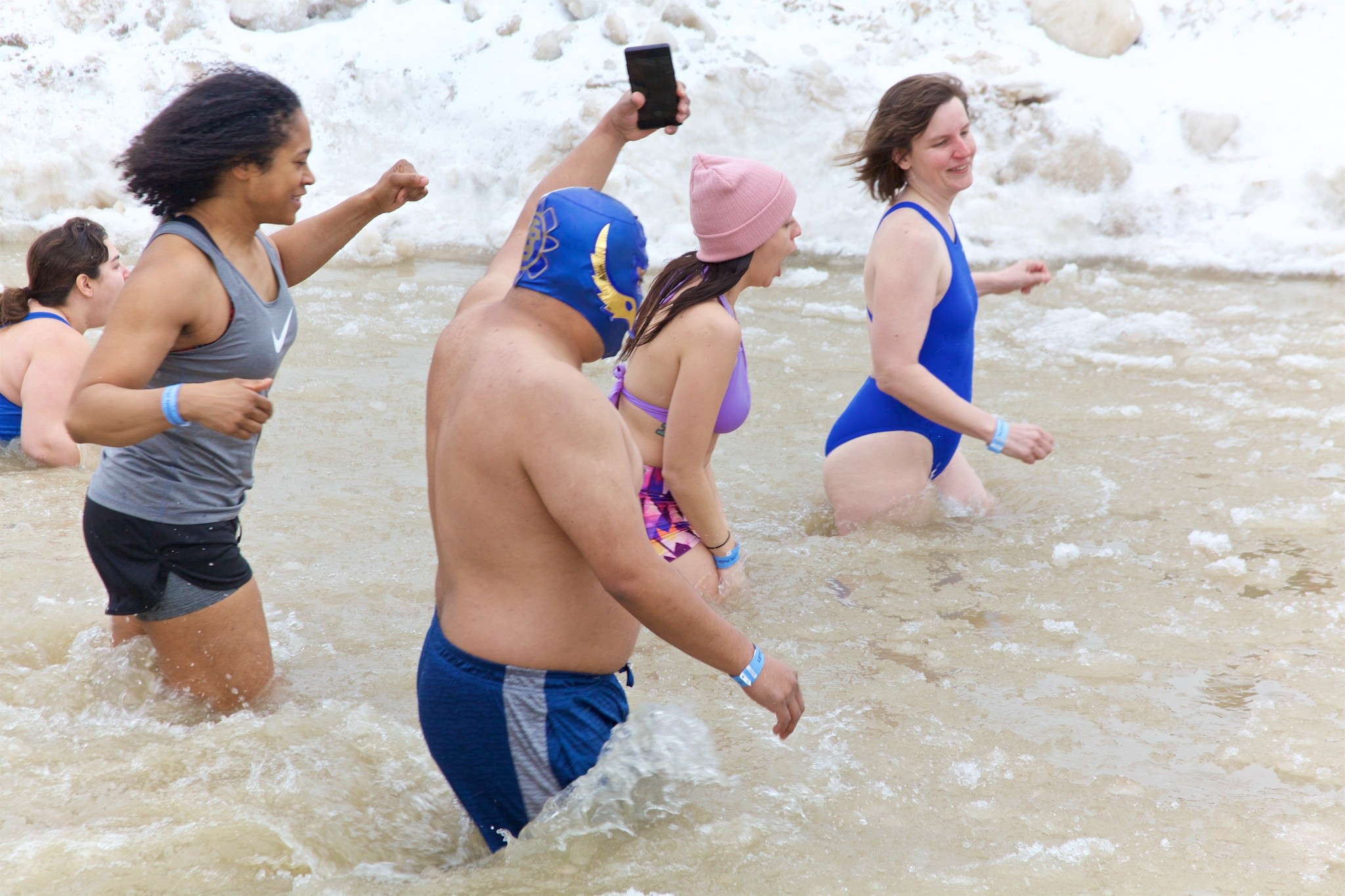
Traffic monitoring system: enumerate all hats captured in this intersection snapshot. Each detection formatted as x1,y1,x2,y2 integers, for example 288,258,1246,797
513,187,649,360
690,153,797,263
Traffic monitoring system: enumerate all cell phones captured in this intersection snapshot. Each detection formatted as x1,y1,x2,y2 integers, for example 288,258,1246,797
625,43,683,130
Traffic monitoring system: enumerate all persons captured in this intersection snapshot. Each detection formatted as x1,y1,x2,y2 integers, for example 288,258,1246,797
418,81,805,853
608,152,801,593
64,61,429,716
824,71,1054,535
0,216,130,468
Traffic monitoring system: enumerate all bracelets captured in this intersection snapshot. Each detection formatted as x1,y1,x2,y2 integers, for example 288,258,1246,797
706,530,730,549
730,641,764,688
987,417,1008,453
161,384,191,426
714,540,740,568
